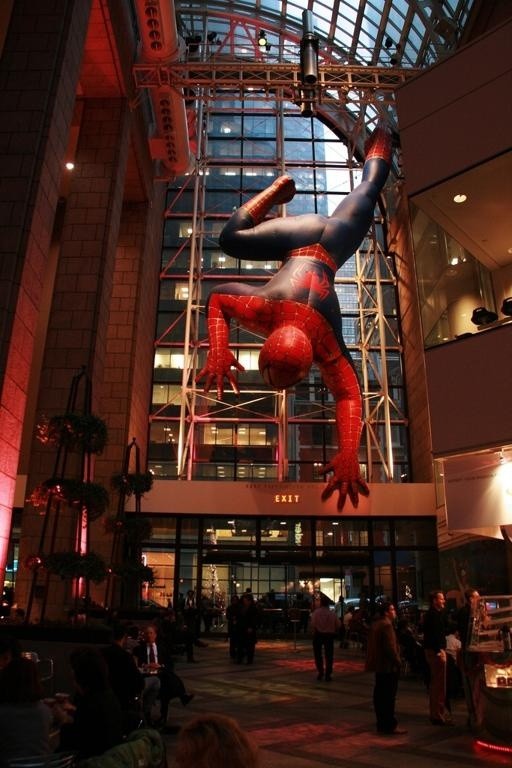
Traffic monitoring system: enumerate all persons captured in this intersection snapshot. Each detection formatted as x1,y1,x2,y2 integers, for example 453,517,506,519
178,590,266,663
295,584,341,681
1,624,173,768
358,586,482,739
192,118,401,512
174,713,262,767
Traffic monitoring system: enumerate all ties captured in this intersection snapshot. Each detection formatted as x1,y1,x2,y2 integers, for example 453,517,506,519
149,644,155,663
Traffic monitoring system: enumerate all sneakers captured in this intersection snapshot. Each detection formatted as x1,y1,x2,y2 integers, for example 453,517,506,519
393,727,408,736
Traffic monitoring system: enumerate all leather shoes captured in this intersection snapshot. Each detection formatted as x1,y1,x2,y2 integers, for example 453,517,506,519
182,694,195,706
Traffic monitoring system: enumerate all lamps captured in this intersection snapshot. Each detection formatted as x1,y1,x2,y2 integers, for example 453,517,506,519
207,31,217,42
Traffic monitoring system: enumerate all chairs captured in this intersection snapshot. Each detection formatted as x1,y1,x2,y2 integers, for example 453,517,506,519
0,728,163,768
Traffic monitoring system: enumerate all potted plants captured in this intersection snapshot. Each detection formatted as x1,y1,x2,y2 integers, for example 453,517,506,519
25,409,157,587
